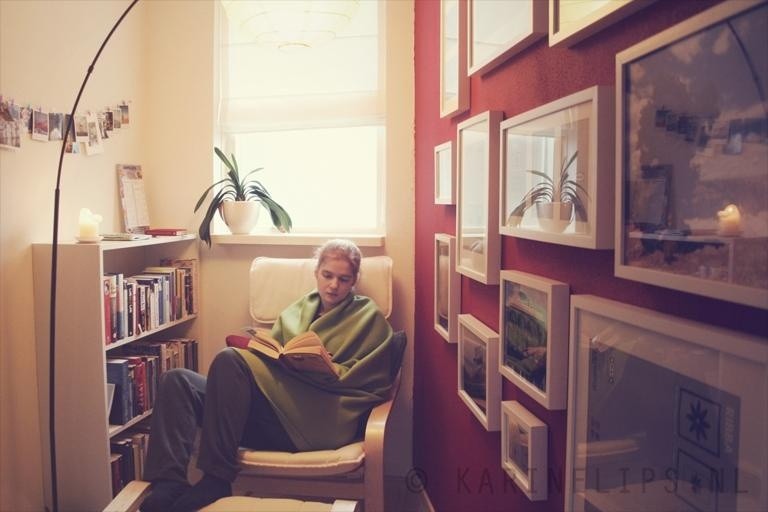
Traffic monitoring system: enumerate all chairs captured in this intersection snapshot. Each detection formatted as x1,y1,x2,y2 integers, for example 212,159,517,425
221,256,408,510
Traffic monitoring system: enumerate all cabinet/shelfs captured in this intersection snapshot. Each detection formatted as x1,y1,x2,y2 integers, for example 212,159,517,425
33,234,204,510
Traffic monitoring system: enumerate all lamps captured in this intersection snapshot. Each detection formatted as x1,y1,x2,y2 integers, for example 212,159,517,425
221,256,408,510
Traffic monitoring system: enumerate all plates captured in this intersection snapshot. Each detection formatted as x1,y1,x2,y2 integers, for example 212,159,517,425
33,234,204,510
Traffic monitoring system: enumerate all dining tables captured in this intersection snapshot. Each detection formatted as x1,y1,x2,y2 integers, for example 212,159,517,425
195,145,294,250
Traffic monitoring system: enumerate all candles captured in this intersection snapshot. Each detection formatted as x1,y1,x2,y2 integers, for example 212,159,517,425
77,207,100,238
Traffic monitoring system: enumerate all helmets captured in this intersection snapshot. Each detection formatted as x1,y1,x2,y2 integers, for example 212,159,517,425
74,236,104,242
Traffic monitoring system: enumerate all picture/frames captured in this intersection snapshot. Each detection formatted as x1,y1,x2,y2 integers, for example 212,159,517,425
498,270,570,410
439,1,469,120
457,314,501,432
499,85,614,251
466,0,547,78
501,402,548,501
564,294,767,512
614,3,768,307
434,233,461,342
433,140,457,205
455,110,504,285
550,0,654,50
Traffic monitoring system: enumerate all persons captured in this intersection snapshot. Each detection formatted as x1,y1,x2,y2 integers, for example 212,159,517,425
140,238,395,511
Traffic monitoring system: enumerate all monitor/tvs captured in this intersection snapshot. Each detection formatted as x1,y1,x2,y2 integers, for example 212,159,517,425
99,233,152,241
247,331,340,380
146,230,187,236
102,259,197,500
116,164,150,234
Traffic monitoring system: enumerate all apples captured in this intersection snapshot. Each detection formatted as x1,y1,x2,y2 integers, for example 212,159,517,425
77,207,100,238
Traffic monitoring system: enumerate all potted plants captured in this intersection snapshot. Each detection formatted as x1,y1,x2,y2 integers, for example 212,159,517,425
195,145,294,250
